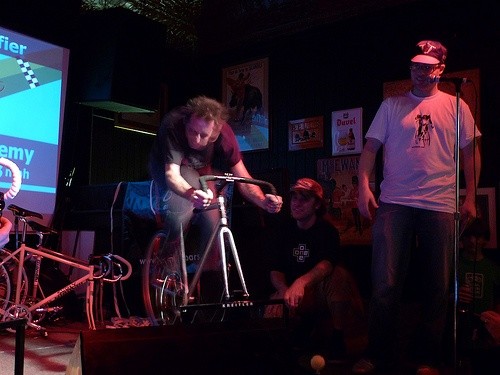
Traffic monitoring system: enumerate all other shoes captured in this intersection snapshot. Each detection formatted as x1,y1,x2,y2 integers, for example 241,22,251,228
416,365,440,375
352,358,375,375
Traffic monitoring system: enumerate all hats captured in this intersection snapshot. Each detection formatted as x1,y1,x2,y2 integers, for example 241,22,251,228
289,179,323,199
411,40,447,64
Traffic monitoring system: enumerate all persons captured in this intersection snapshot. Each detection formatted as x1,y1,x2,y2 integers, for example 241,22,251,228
263,178,366,365
0,217,12,249
480,310,500,346
353,39,482,375
150,95,283,302
456,216,500,374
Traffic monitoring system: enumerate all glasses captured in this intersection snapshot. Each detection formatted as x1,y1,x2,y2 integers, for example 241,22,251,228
409,65,439,73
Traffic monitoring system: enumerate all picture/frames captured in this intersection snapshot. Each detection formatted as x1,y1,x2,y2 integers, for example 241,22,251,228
286,114,326,152
458,187,497,249
220,54,272,154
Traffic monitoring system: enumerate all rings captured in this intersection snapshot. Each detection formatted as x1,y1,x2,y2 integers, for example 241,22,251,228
485,319,488,324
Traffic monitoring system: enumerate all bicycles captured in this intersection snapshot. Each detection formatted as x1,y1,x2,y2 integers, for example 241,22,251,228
141,172,283,334
0,204,135,338
